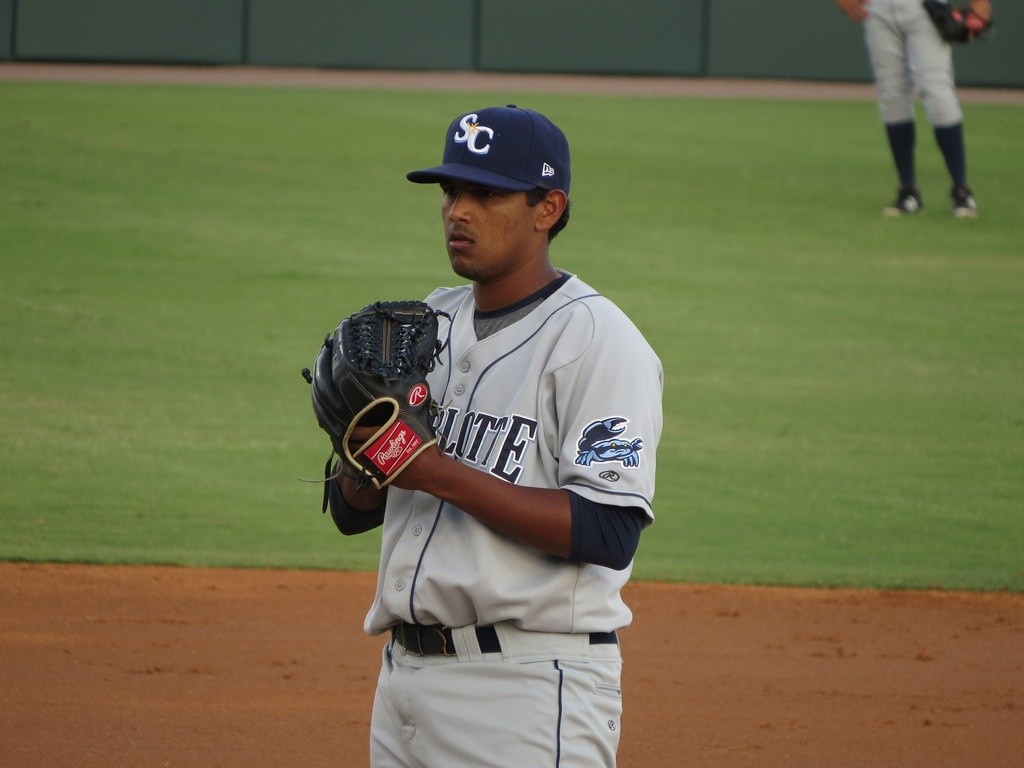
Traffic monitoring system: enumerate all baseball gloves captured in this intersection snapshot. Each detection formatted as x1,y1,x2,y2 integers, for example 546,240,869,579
310,303,438,489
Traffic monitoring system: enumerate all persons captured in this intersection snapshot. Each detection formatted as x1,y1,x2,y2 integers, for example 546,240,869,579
836,0,993,218
303,107,665,768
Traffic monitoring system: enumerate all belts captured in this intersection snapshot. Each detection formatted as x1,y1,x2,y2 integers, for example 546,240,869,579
391,618,618,658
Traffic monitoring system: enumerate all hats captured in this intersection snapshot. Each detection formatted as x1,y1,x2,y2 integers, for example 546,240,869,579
406,104,571,195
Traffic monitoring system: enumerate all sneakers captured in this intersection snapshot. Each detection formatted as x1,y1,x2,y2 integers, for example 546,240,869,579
951,184,977,219
883,186,922,216
